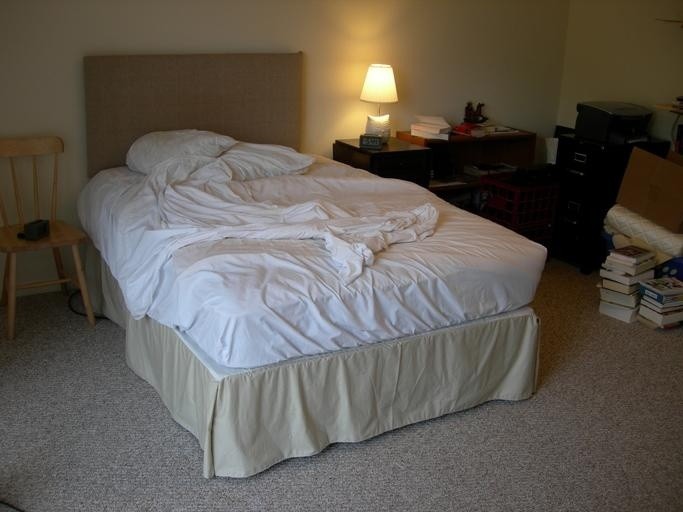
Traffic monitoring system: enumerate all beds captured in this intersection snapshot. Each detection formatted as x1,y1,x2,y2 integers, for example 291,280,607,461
83,52,549,477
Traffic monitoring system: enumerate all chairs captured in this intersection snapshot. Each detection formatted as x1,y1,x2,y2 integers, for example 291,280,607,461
0,136,96,341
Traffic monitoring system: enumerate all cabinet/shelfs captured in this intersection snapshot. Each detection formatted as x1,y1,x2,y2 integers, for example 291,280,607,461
396,125,538,206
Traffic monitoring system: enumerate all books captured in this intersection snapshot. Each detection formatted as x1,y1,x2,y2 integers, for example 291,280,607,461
597,245,683,330
409,114,518,188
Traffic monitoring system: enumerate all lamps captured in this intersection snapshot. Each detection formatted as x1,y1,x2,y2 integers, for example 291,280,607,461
359,64,399,144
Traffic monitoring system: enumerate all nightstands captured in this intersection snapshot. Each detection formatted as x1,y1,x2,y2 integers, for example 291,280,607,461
331,137,432,189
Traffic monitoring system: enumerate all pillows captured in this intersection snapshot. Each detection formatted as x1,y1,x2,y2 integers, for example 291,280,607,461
123,128,317,177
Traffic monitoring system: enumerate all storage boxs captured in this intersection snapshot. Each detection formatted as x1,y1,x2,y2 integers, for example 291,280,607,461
553,96,683,275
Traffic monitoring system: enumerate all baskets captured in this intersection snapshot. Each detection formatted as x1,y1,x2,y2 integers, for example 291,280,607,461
479,176,560,245
